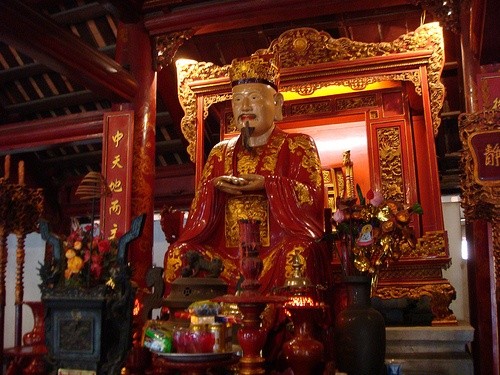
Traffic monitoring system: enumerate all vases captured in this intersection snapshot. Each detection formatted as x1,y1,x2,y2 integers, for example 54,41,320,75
335,277,386,375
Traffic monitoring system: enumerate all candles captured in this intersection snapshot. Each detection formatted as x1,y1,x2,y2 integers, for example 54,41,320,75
5,155,10,177
18,161,26,186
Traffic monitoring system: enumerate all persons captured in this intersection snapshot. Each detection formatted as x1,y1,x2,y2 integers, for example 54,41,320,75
161,50,325,366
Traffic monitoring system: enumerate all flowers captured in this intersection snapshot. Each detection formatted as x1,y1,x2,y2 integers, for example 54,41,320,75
327,184,423,278
49,223,119,291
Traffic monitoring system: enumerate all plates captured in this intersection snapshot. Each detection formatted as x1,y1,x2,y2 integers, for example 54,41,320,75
158,352,232,362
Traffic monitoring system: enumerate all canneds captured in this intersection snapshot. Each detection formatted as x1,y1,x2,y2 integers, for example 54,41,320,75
190,323,206,338
207,323,225,353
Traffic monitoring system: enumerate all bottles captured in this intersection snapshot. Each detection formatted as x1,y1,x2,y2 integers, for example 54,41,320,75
283,306,324,375
20,302,48,375
